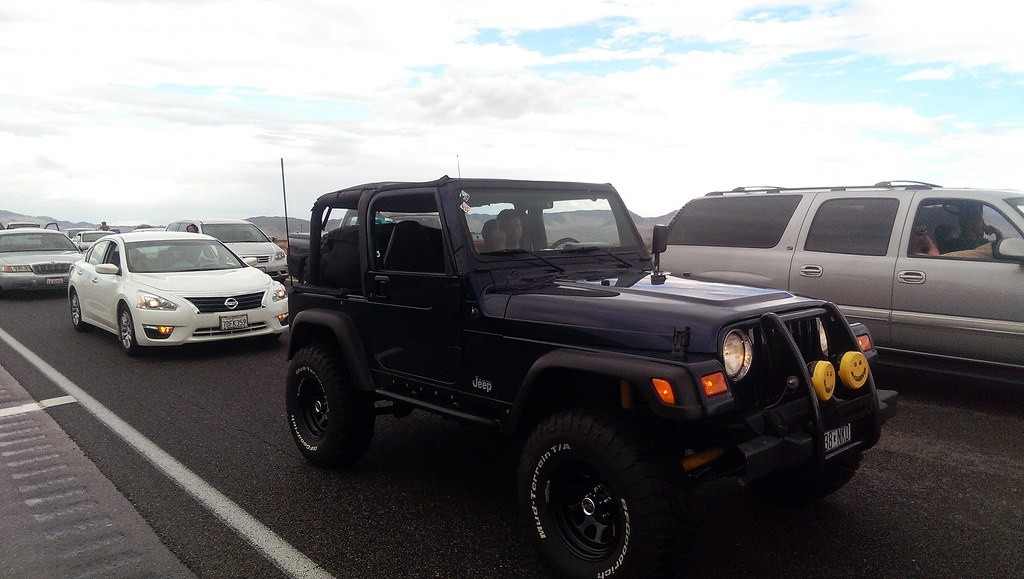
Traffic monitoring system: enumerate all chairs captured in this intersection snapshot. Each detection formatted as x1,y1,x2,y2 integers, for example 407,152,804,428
382,221,445,273
934,223,958,246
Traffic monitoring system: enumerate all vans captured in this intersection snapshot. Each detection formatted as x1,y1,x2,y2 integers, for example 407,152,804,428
654,179,1024,389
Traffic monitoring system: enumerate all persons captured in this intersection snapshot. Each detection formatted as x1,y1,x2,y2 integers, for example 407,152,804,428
99,222,110,231
186,225,198,233
956,206,1004,251
912,224,940,256
491,208,522,252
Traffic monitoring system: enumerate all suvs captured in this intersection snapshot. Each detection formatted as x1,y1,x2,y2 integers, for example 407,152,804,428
282,177,899,579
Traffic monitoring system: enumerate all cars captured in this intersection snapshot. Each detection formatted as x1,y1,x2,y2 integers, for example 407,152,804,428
70,231,116,253
166,219,289,286
0,227,84,292
67,230,289,352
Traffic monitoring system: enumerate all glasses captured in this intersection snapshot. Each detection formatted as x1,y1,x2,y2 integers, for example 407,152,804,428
502,224,524,231
